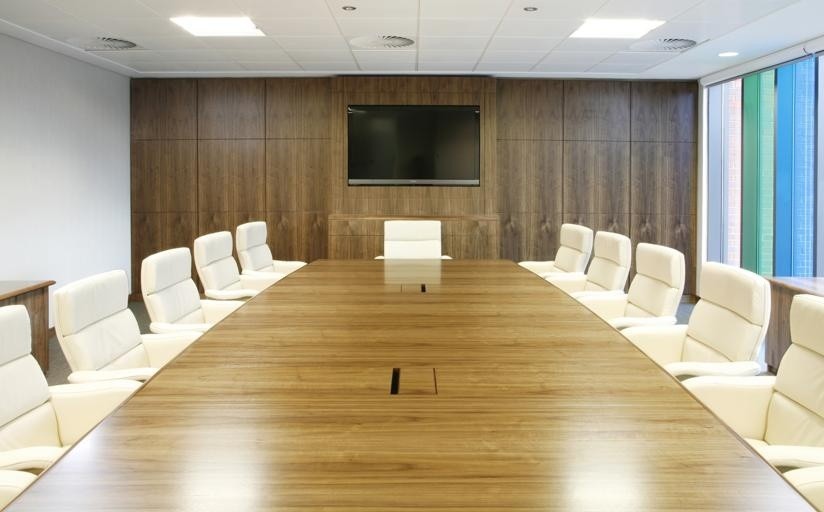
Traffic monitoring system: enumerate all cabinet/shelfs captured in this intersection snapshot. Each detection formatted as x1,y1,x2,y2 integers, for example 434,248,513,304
1,279,57,374
761,274,824,373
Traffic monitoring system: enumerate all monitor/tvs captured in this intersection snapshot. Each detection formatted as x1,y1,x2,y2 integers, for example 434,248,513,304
347,104,480,186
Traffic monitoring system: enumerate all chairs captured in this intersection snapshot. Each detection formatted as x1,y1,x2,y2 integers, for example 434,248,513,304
0,305,142,468
235,220,308,279
546,231,633,296
139,246,245,333
0,469,39,512
575,242,685,328
193,230,284,300
781,467,824,511
680,292,824,468
51,269,205,381
516,224,593,284
618,262,773,377
373,220,452,259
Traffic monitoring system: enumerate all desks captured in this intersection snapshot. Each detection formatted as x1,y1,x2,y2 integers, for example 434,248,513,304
1,257,824,512
